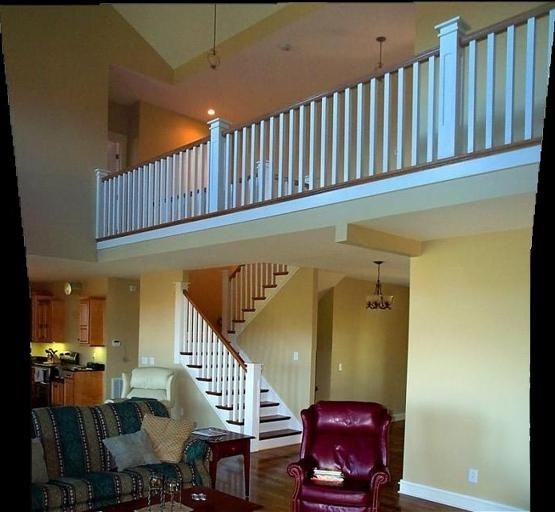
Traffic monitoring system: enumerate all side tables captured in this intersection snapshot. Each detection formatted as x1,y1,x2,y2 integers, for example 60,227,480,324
191,427,255,497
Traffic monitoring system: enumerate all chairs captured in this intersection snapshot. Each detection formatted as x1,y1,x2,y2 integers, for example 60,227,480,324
106,366,177,417
287,401,392,512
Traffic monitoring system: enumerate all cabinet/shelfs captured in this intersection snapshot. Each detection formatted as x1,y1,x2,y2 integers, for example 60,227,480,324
78,296,105,347
32,363,103,406
31,291,66,342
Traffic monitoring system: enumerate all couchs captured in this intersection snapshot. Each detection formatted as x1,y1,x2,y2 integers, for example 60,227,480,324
30,399,213,512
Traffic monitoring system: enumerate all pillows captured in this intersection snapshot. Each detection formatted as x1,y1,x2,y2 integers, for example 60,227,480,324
31,436,48,484
141,413,193,464
103,429,161,473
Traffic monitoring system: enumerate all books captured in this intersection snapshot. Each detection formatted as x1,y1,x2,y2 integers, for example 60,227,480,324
310,468,344,488
193,429,226,437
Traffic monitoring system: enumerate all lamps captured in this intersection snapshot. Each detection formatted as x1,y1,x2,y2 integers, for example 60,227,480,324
365,260,395,310
